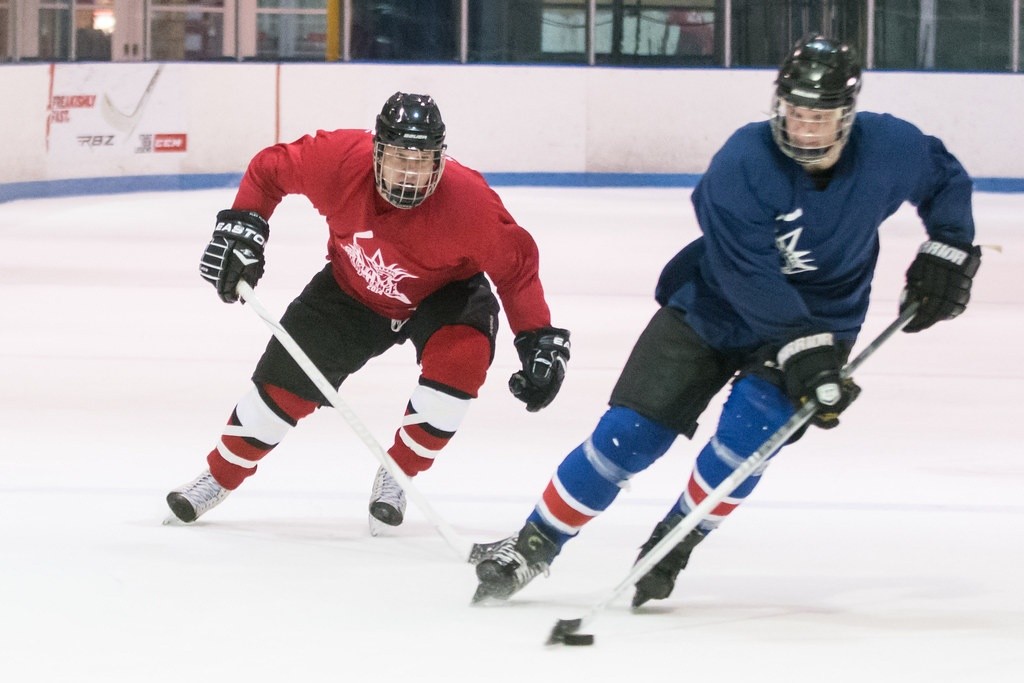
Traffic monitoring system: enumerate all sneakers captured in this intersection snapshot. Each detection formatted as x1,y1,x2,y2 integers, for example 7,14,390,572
632,509,708,608
368,462,408,535
162,468,230,525
471,523,562,605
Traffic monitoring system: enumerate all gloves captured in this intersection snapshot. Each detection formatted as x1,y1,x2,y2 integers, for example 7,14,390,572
898,231,983,334
507,327,573,414
199,206,270,305
768,327,863,429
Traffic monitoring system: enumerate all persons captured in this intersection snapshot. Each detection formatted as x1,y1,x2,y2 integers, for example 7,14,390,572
162,95,570,534
472,32,975,605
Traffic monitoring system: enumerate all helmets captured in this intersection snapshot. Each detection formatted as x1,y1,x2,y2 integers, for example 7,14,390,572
768,33,863,173
373,92,447,210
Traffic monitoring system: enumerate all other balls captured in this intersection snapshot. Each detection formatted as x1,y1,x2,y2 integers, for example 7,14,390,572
564,631,595,648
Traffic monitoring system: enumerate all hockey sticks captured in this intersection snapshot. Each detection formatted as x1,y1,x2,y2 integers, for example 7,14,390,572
545,297,922,647
236,277,412,501
